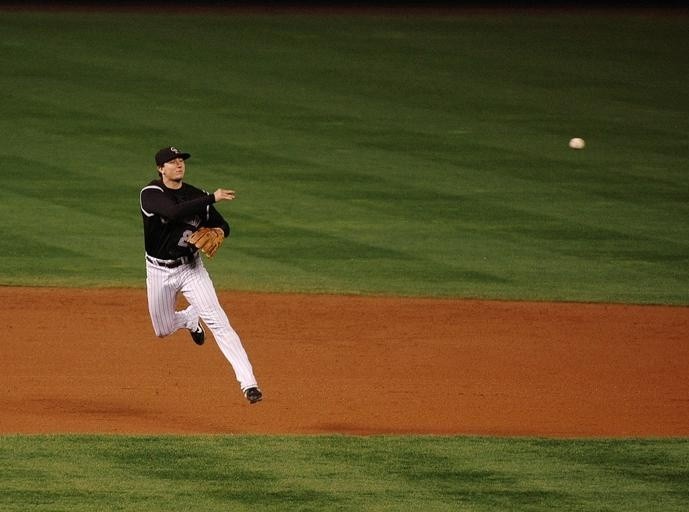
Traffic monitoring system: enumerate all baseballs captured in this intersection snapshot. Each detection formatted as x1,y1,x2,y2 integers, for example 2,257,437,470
569,138,584,149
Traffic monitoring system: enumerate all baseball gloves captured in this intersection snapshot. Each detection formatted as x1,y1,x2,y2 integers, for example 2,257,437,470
186,227,224,259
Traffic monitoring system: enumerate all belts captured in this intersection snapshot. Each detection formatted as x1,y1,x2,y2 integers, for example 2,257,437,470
146,252,201,269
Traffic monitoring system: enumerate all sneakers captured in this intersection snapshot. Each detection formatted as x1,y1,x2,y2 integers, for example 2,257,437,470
243,387,263,404
189,321,207,346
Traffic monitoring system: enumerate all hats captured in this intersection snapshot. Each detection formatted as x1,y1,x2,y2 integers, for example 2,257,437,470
154,146,192,166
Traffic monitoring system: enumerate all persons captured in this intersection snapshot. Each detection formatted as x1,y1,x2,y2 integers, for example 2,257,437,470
139,145,263,403
